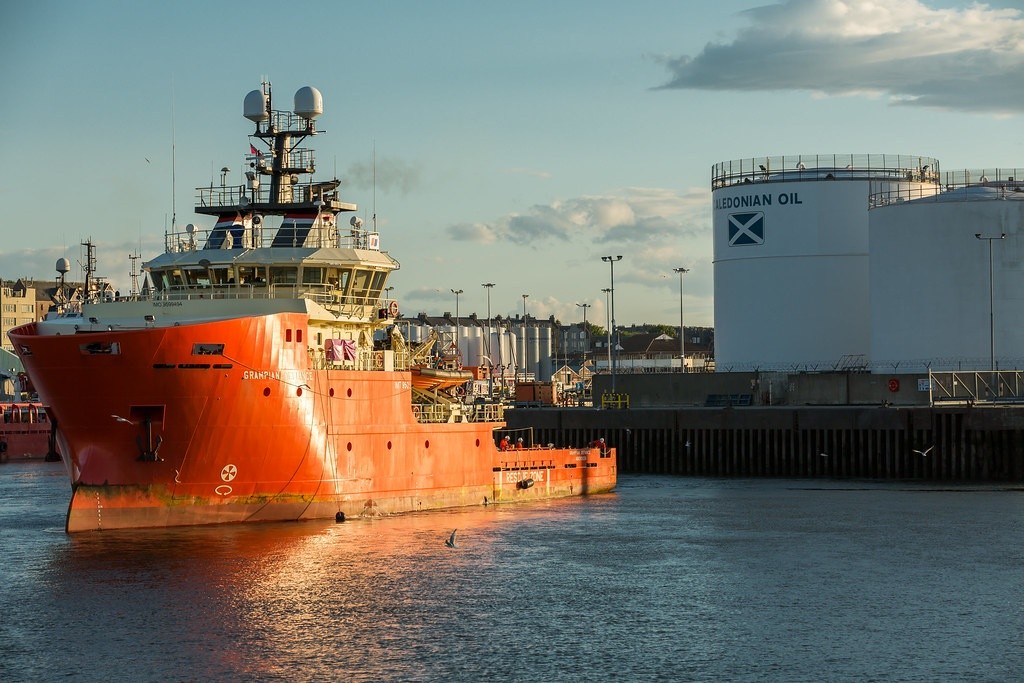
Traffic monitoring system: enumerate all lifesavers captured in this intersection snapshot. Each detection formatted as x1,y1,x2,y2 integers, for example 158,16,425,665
375,354,383,367
390,301,399,316
457,387,464,395
558,392,564,401
413,407,419,419
888,378,899,392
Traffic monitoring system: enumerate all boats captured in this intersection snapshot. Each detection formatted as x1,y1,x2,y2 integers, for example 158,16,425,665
7,77,618,533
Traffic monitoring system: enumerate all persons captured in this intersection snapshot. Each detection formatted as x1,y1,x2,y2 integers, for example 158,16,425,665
588,438,606,449
242,274,262,286
516,438,523,451
500,435,513,452
250,143,258,154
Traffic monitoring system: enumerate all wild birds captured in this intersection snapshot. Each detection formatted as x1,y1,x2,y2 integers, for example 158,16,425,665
445,529,458,547
913,445,934,457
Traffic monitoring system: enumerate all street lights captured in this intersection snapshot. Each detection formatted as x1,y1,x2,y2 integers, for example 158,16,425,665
576,303,591,380
600,287,614,373
673,268,690,371
481,283,495,360
384,286,394,298
601,254,623,393
451,289,463,355
522,294,530,382
